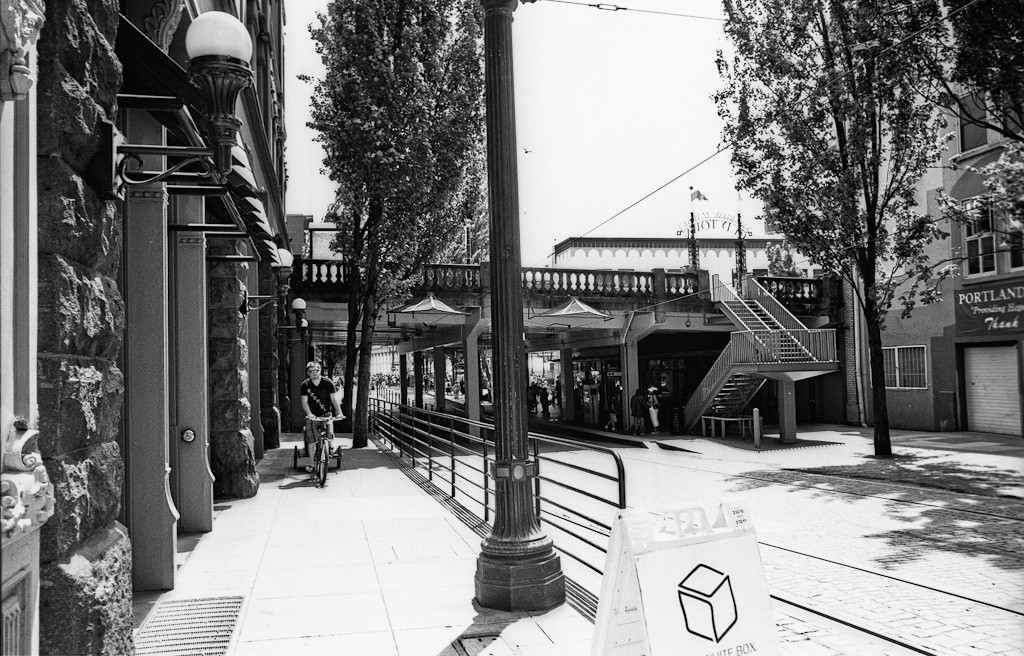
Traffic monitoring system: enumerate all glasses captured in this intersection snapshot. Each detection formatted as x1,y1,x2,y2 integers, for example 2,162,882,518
308,370,320,374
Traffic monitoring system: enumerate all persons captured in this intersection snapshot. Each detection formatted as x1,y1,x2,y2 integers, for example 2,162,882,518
631,386,660,435
300,361,344,472
370,373,434,393
529,376,561,413
486,378,491,402
604,393,618,433
445,374,465,399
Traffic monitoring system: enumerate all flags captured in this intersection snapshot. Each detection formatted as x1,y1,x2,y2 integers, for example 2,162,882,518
689,186,708,202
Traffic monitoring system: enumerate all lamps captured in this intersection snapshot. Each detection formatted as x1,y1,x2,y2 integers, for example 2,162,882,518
277,296,308,338
98,9,257,204
242,247,300,314
283,317,308,357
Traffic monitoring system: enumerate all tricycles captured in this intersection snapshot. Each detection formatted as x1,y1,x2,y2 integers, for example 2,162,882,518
292,416,347,485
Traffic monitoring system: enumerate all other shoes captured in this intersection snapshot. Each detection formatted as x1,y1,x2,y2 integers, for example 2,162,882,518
605,427,609,432
651,432,659,434
640,432,645,435
633,431,638,435
329,446,334,458
612,430,618,433
306,464,314,472
